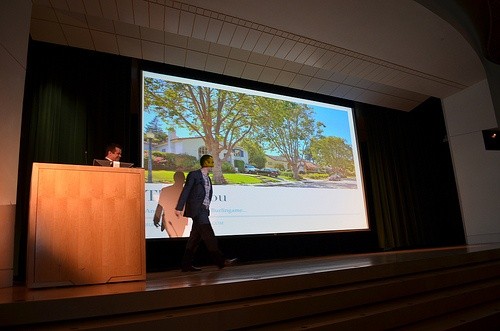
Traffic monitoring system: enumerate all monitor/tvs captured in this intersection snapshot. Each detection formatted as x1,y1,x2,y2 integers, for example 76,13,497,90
93,159,134,169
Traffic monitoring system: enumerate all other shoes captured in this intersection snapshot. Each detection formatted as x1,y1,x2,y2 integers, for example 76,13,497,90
219,258,237,269
181,265,202,272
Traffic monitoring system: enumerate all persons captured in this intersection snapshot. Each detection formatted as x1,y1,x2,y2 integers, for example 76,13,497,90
103,143,122,162
175,154,238,273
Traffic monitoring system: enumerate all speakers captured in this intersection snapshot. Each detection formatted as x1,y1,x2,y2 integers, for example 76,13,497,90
482,128,500,150
